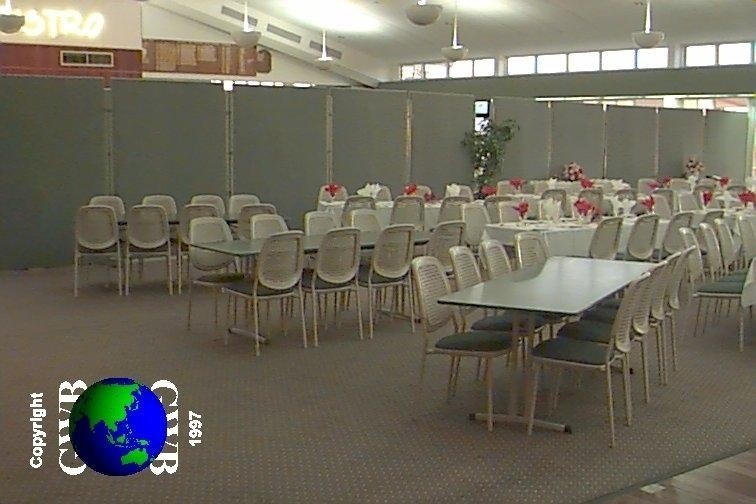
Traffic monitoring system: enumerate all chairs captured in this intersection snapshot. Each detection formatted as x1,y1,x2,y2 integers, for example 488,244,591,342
73,179,756,447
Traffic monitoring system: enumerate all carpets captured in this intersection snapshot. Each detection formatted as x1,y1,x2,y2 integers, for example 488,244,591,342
1,257,756,503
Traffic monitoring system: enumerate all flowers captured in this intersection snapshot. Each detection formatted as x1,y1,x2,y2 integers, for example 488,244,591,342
563,162,584,180
683,153,704,176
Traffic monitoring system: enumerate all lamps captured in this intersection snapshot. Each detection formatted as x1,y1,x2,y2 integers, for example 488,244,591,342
233,0,261,48
406,0,442,26
440,0,468,61
0,0,25,34
314,29,333,70
632,0,664,48
